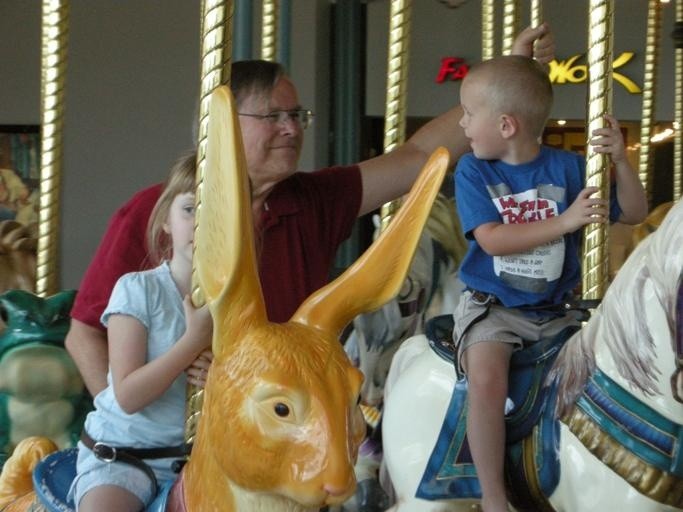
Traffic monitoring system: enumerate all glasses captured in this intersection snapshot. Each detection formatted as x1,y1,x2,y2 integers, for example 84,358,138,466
239,108,317,129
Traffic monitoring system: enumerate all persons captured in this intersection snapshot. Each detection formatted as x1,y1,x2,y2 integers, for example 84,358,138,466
63,20,558,398
451,55,650,512
66,150,212,512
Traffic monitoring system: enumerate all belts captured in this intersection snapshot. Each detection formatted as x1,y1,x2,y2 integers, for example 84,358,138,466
454,288,602,382
74,431,198,495
